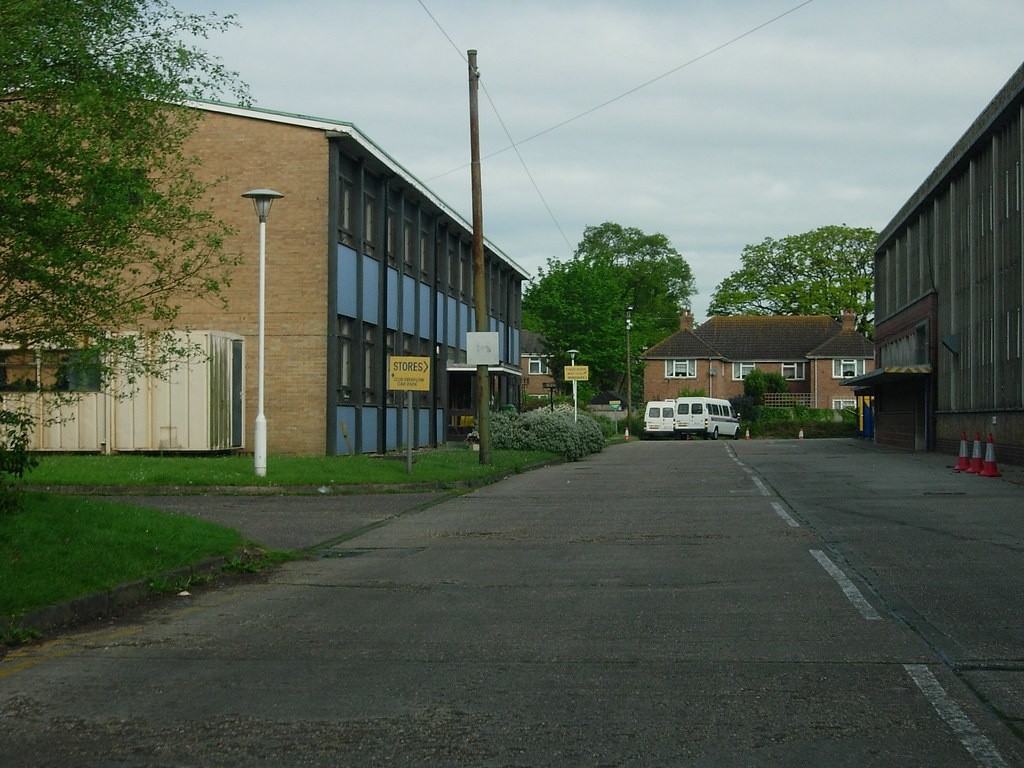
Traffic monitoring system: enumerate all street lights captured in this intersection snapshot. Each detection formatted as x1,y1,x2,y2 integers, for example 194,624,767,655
566,350,580,423
240,189,287,477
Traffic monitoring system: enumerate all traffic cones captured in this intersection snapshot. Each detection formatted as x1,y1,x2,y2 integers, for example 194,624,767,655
979,432,1001,477
952,432,971,470
966,432,985,474
624,427,630,443
798,428,803,440
745,427,751,440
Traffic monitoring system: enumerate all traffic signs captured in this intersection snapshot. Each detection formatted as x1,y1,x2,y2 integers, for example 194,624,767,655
387,352,431,393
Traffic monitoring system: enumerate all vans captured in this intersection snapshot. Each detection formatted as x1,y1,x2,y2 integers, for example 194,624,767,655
642,399,688,440
672,395,742,441
